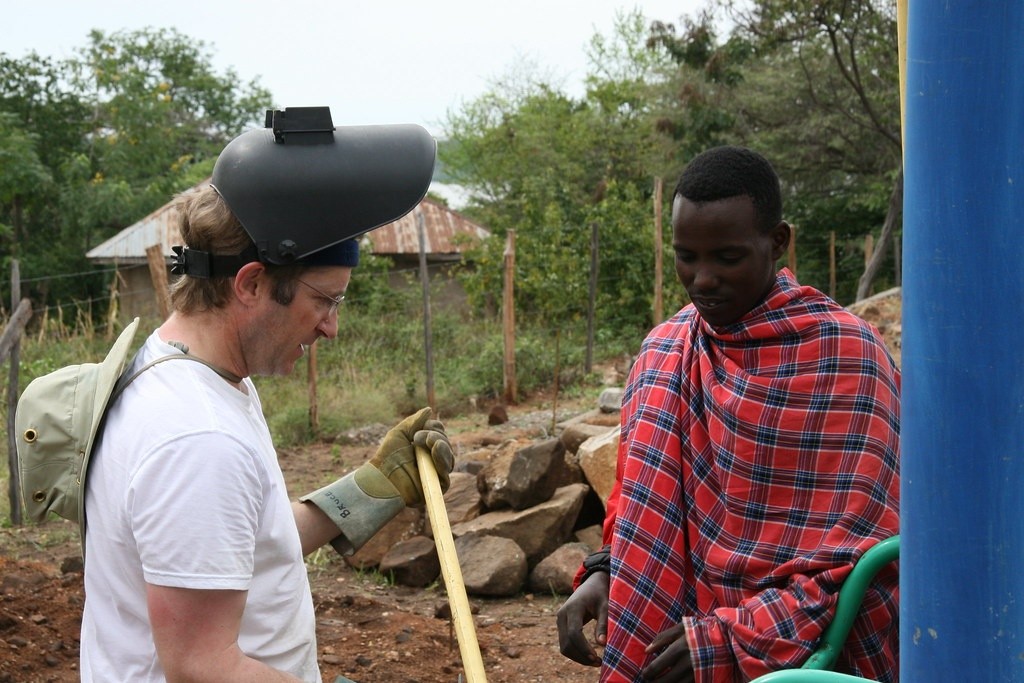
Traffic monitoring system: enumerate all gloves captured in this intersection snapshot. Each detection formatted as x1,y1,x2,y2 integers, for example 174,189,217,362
300,407,455,558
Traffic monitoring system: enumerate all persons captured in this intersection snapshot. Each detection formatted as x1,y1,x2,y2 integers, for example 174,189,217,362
555,148,900,683
79,107,456,682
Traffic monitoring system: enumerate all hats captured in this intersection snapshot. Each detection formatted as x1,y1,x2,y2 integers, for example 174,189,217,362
16,318,144,560
